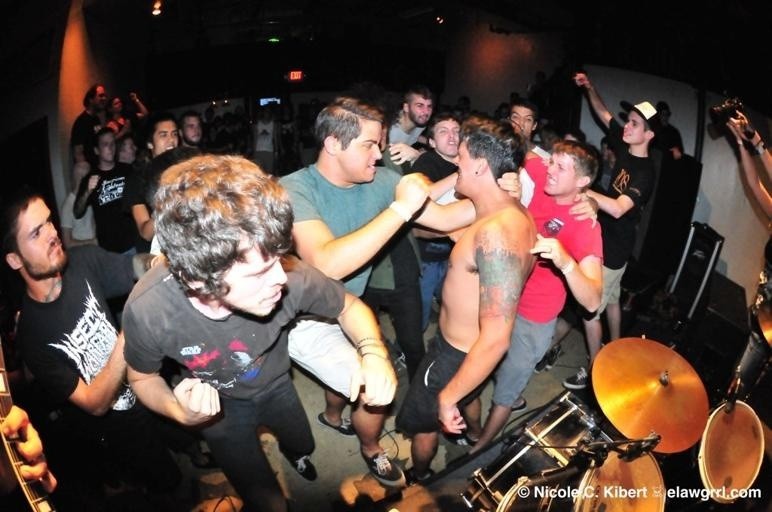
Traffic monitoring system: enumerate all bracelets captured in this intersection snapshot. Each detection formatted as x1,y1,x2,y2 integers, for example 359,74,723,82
753,139,765,148
753,144,770,154
735,140,744,147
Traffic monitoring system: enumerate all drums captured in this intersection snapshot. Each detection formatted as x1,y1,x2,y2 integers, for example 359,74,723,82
672,399,766,506
492,439,667,512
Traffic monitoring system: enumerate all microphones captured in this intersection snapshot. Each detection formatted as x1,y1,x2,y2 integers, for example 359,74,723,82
622,437,660,463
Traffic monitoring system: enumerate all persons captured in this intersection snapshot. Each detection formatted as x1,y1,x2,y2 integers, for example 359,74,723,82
272,92,526,491
121,151,399,512
62,83,685,382
724,110,771,394
392,116,543,489
1,193,200,512
442,139,606,469
564,70,660,390
0,403,59,500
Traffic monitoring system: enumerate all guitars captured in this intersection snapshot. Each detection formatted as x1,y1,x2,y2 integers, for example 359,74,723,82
0,335,55,512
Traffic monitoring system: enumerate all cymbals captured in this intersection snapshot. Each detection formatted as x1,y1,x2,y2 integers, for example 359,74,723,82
591,337,712,455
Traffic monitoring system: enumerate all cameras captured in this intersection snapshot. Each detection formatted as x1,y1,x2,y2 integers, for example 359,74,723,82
708,94,745,122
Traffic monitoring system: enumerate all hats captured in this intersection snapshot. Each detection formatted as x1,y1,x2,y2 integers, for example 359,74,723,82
619,99,662,131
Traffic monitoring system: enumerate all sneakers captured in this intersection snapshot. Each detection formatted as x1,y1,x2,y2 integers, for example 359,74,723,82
292,455,319,481
561,367,589,389
358,442,406,487
192,454,219,468
511,398,527,413
318,411,356,435
534,345,562,374
408,467,435,481
443,430,478,446
393,348,407,367
